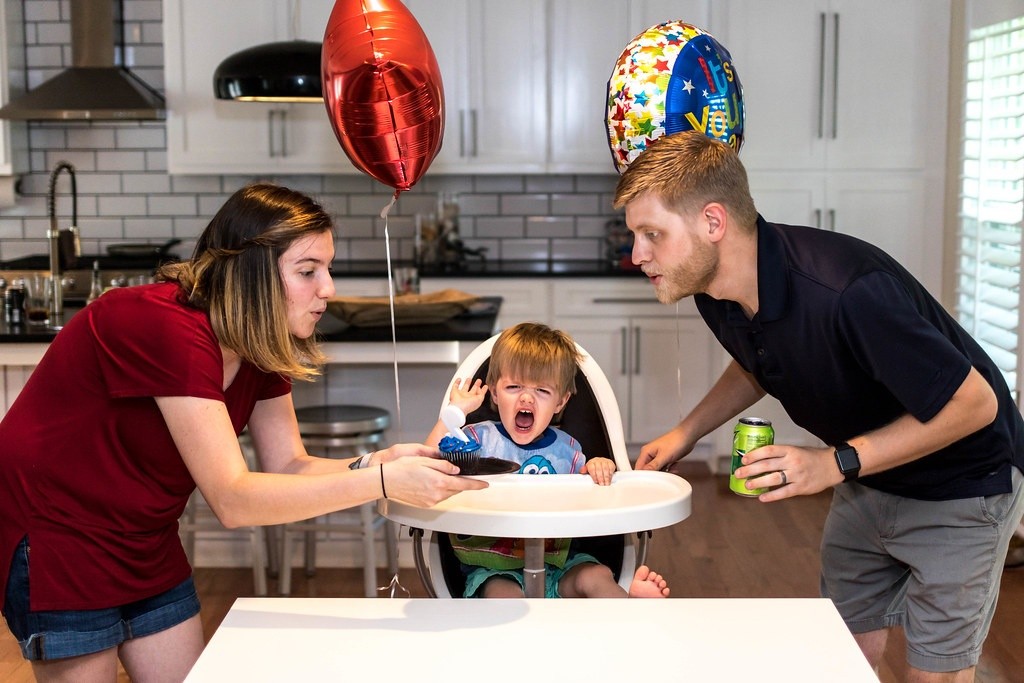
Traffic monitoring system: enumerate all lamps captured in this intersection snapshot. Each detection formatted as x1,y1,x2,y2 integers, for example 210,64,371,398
213,0,324,104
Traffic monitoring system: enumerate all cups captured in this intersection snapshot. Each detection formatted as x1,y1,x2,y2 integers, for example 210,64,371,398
24,277,50,324
128,276,154,288
394,266,420,296
415,213,439,265
439,192,458,236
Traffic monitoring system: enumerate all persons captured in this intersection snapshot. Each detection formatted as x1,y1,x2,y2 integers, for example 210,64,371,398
421,321,672,598
615,128,1024,683
0,180,487,682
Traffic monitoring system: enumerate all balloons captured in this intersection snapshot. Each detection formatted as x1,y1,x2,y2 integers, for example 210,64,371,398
321,1,445,193
604,20,746,179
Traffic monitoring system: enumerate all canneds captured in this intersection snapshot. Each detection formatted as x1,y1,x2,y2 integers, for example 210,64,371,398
5,286,25,324
729,417,775,497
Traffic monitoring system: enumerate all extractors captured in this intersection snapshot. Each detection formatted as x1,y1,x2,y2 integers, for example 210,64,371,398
0,0,166,121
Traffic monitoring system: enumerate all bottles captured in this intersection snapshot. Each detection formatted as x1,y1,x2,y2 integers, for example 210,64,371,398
87,270,103,306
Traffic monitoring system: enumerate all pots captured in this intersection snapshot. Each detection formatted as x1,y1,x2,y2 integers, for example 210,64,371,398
107,237,181,262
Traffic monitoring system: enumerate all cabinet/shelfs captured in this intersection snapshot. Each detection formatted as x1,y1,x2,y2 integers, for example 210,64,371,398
0,0,932,476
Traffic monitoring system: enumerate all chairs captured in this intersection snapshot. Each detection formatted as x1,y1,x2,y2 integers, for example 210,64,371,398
377,329,694,599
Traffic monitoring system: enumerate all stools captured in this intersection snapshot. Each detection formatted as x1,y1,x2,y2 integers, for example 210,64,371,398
279,405,398,598
174,432,279,597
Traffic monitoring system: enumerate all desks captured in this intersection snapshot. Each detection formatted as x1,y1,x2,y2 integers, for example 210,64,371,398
179,598,882,683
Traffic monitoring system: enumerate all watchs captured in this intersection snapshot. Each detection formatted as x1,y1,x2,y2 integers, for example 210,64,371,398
830,438,862,481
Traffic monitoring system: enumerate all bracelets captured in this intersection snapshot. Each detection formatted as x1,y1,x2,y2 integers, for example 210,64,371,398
349,446,374,471
379,462,388,499
435,402,467,431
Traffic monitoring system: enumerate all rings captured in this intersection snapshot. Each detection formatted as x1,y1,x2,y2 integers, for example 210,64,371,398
778,469,788,484
